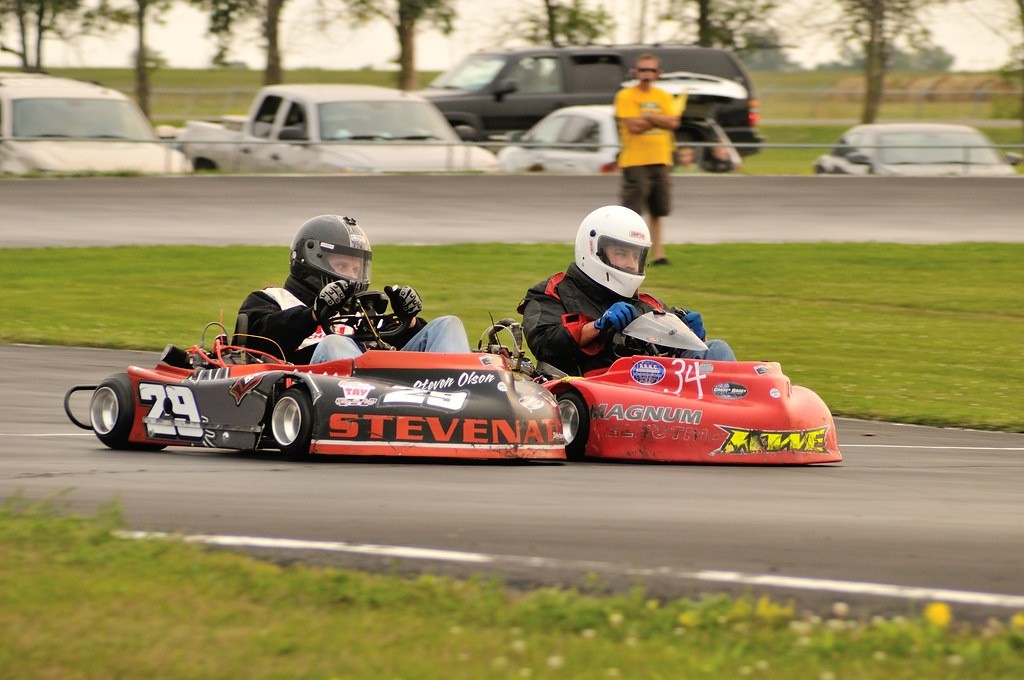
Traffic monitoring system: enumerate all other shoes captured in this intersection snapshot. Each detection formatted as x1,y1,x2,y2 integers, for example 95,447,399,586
650,258,671,266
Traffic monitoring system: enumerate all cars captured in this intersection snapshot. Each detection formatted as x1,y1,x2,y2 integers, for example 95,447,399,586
0,71,198,174
814,121,1024,178
485,70,748,184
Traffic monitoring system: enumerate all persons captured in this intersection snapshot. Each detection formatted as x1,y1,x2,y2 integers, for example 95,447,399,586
232,215,472,365
615,54,678,264
519,205,737,376
671,145,707,175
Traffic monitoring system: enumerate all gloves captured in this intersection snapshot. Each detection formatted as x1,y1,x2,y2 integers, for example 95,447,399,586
312,280,348,325
681,308,706,343
384,285,423,326
594,301,638,335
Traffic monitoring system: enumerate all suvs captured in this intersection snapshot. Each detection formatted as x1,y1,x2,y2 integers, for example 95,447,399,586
426,46,765,156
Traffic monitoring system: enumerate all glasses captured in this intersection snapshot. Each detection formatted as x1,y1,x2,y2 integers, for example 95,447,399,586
635,68,659,73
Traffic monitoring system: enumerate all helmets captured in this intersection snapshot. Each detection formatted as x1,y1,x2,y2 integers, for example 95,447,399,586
575,204,653,298
289,215,372,306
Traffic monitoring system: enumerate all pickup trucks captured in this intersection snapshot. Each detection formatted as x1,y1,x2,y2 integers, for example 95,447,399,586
181,81,505,178
